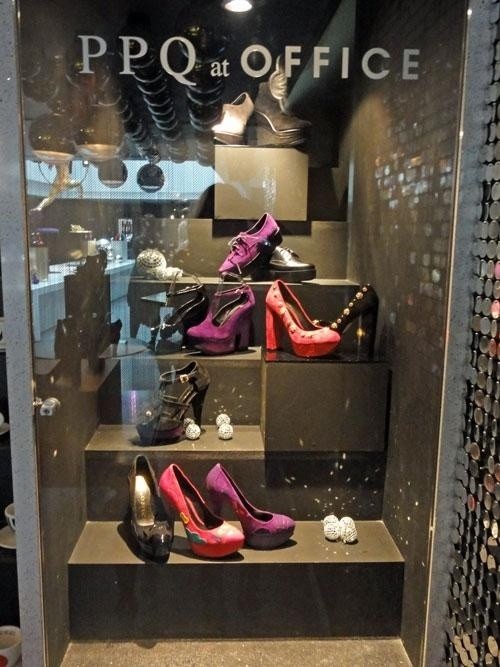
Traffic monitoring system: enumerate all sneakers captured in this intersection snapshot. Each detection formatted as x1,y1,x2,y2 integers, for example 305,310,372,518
218,212,316,281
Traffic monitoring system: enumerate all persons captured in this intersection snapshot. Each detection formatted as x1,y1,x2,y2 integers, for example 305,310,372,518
177,206,192,249
160,184,272,346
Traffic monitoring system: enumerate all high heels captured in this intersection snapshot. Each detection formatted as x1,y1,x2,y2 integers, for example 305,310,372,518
136,360,210,440
265,280,342,358
205,464,296,549
147,283,256,355
159,463,247,559
312,283,379,358
210,92,255,146
254,55,312,147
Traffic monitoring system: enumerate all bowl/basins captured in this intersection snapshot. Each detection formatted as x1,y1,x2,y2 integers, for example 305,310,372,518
0,624,21,666
4,502,16,533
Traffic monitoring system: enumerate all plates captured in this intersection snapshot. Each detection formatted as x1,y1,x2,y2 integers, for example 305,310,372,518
0,525,16,550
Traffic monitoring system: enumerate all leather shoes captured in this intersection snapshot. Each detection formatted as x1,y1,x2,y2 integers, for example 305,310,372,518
127,453,174,561
